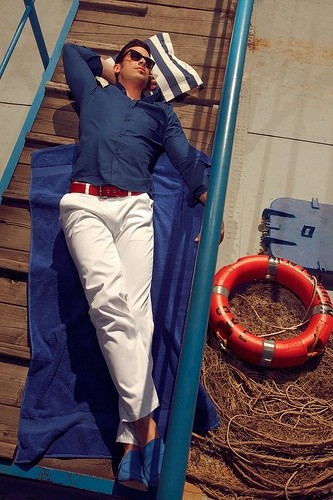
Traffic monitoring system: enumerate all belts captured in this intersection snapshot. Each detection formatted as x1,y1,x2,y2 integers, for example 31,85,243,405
68,183,144,198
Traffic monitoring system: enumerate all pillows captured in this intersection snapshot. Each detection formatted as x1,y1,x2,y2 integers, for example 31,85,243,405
96,33,203,103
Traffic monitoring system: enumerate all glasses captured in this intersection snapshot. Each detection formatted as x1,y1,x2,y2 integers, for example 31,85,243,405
119,48,155,71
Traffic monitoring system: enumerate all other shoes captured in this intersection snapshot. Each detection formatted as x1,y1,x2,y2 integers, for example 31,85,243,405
139,438,167,488
117,449,149,493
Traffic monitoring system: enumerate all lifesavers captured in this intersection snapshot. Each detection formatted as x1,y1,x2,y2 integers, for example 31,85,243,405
207,254,333,370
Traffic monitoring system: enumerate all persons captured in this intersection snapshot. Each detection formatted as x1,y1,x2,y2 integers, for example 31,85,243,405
61,39,225,491
